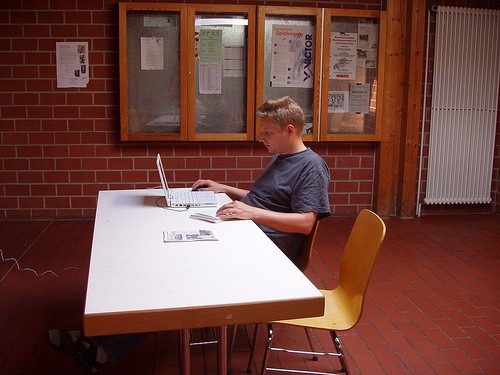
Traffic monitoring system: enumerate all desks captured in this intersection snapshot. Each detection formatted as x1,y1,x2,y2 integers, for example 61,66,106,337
83,190,324,375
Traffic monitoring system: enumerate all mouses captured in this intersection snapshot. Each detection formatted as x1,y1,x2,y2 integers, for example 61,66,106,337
192,185,207,191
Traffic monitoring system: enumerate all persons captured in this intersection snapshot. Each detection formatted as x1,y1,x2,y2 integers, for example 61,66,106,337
45,95,332,375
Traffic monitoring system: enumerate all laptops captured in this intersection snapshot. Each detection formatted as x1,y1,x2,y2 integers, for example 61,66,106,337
156,153,218,208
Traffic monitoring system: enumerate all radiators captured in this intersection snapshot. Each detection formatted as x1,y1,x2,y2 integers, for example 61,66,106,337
424,5,500,205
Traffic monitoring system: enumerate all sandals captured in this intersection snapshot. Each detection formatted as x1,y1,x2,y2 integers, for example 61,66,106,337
47,327,96,355
62,332,104,375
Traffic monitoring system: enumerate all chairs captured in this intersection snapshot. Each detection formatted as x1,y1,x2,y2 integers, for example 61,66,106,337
227,217,318,374
248,209,386,375
